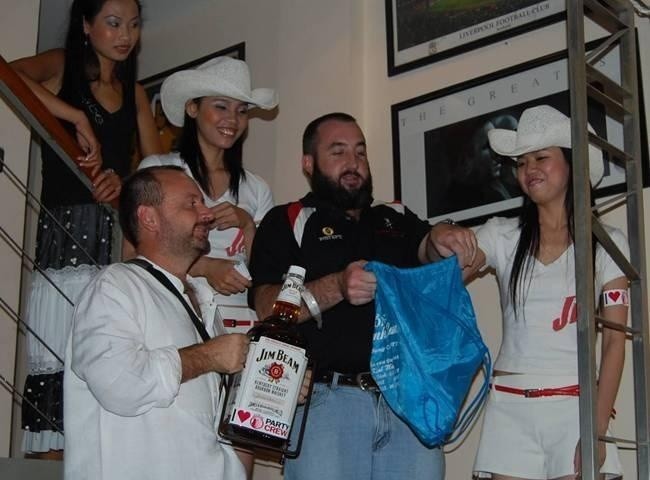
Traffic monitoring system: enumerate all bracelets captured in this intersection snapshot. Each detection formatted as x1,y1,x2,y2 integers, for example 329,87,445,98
436,217,457,227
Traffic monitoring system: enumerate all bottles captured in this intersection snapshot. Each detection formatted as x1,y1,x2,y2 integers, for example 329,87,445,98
218,265,318,459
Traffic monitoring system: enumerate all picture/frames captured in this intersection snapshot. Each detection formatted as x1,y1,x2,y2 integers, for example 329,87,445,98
137,42,245,146
391,27,650,227
385,0,567,77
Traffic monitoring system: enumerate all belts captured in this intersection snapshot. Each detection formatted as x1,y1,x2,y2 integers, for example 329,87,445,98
488,380,599,397
312,372,379,390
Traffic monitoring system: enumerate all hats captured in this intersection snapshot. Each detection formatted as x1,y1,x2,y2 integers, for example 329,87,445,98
487,104,597,158
160,57,280,128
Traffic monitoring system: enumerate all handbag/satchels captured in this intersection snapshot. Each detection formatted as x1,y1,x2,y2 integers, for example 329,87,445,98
362,253,492,451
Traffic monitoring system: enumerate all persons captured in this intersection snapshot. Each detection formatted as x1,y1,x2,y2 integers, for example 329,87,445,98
443,101,635,479
58,162,313,480
243,110,481,480
8,0,167,459
120,55,276,341
